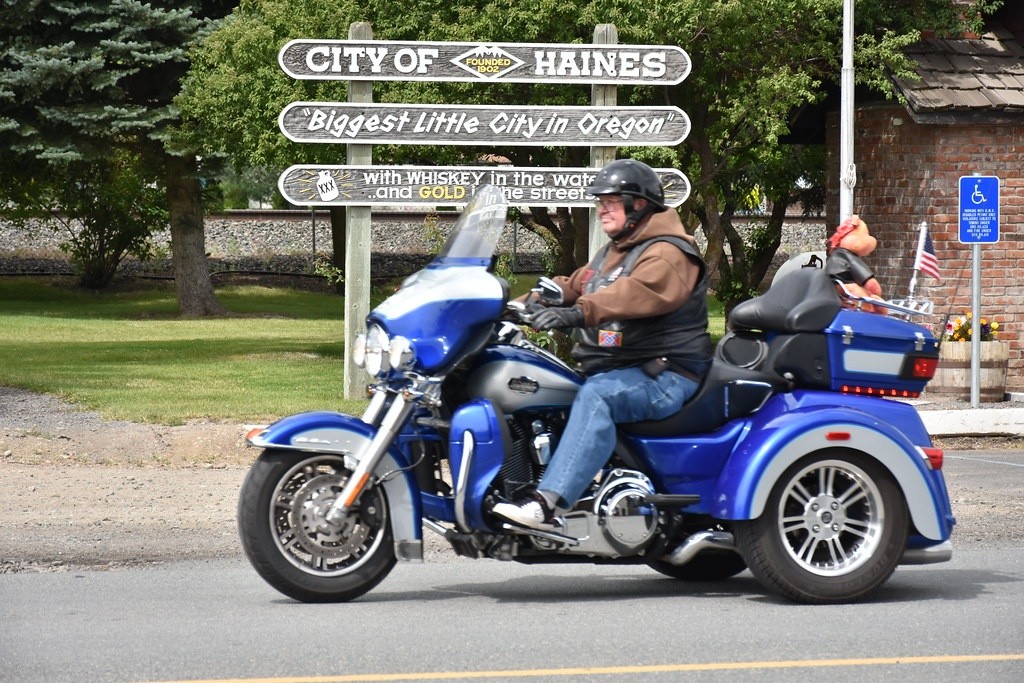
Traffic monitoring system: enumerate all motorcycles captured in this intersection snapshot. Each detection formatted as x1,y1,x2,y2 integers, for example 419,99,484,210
237,180,955,607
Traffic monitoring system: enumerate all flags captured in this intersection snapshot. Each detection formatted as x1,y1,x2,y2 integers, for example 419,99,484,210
920,229,940,281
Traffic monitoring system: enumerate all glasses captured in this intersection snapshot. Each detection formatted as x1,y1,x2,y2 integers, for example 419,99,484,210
594,199,622,206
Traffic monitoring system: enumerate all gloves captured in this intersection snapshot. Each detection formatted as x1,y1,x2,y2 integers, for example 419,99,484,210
528,306,585,331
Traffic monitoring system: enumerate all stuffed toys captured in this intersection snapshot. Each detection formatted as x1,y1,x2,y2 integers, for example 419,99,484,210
825,215,890,316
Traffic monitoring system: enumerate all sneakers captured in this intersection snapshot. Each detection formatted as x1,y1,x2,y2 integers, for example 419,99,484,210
492,489,554,531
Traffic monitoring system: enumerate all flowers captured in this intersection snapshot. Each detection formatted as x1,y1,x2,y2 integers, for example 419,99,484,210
926,312,999,340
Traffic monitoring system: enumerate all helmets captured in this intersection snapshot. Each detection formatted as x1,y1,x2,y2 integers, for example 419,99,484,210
588,159,668,210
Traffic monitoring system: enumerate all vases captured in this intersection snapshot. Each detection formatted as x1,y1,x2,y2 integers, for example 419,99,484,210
925,342,1010,401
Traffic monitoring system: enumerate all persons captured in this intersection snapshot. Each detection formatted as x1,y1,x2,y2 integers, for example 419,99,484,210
491,158,711,529
